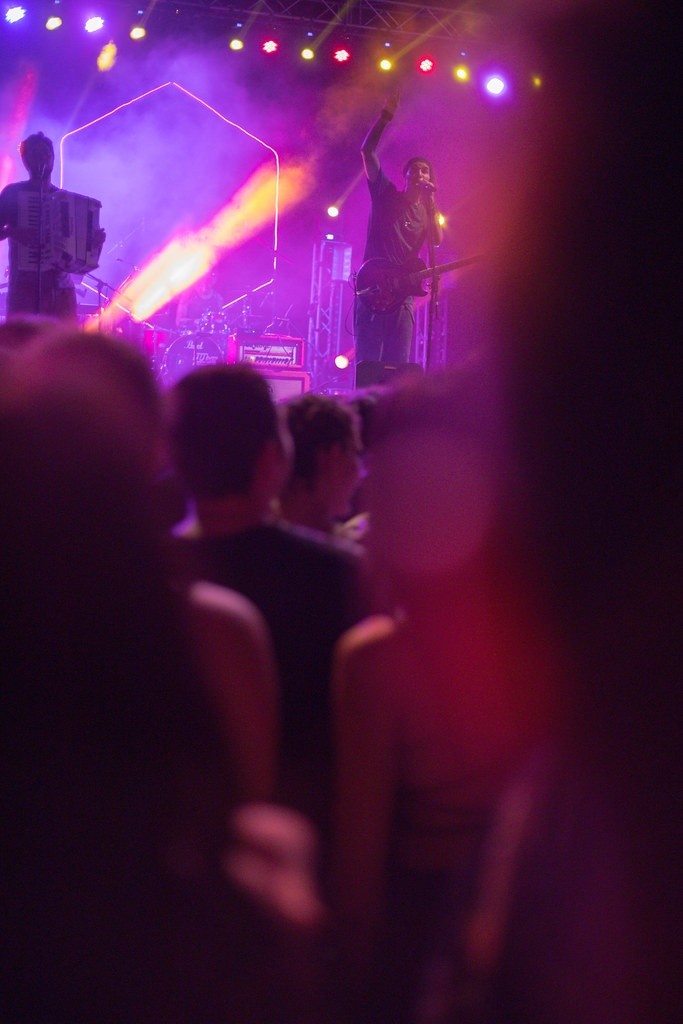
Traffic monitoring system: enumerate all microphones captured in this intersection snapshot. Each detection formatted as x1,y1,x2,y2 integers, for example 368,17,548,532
39,161,46,175
423,184,437,193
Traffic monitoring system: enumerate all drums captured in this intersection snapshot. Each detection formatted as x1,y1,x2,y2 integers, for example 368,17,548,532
162,331,225,380
200,310,230,338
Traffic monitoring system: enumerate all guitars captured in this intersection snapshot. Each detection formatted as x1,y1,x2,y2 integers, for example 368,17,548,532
355,249,494,312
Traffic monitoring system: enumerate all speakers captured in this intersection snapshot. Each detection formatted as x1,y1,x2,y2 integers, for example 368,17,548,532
255,369,311,406
355,360,422,390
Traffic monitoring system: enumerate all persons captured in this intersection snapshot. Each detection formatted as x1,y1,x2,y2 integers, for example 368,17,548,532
0,134,106,318
354,103,443,391
175,270,224,328
0,312,683,1024
